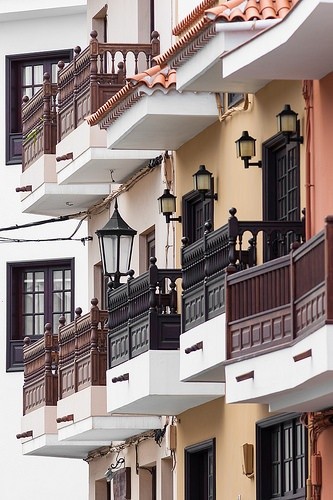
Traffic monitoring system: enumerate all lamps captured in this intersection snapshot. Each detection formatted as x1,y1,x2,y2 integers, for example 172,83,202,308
278,104,303,144
157,188,182,223
192,164,218,202
94,196,139,293
235,131,262,168
